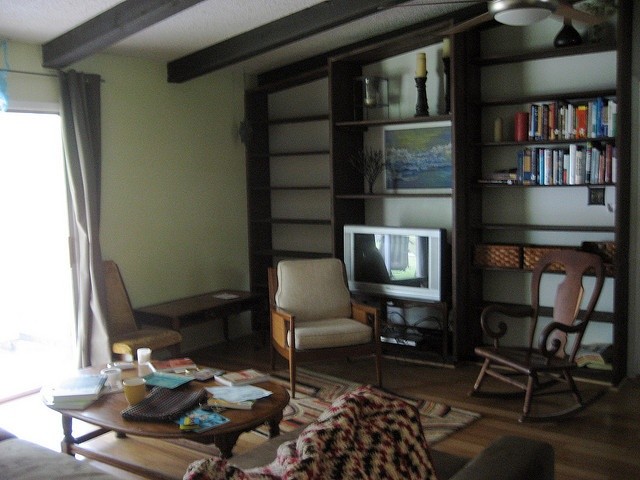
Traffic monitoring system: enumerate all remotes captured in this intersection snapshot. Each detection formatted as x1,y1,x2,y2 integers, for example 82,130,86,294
107,362,134,370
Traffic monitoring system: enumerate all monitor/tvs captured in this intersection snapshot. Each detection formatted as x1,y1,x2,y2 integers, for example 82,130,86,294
343,225,447,304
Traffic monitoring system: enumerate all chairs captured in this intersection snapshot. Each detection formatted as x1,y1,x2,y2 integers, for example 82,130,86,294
101,259,183,355
268,258,383,399
467,250,608,423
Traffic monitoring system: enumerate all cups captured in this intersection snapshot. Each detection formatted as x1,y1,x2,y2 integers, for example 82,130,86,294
102,367,121,384
123,377,146,406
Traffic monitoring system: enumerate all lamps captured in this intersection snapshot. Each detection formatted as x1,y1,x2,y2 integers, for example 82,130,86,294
486,0,557,27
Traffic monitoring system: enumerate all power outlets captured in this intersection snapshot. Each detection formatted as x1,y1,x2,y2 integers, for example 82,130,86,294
332,30,455,226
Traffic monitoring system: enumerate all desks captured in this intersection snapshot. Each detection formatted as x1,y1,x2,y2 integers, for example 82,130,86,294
133,290,257,355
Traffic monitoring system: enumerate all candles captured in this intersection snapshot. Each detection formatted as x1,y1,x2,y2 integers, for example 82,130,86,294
413,53,426,78
441,37,451,56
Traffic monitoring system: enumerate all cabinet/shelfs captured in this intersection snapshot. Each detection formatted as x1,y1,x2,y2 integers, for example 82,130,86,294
240,64,336,335
456,38,628,386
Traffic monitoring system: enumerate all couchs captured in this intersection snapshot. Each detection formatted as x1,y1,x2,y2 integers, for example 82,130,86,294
0,426,555,480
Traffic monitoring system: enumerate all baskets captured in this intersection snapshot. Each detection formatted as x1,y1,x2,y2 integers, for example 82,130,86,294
471,242,522,268
523,247,581,272
581,241,616,276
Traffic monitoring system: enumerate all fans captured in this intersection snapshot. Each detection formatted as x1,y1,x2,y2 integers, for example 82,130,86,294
377,1,610,37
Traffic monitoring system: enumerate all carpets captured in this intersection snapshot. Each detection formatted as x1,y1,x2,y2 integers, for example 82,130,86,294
251,359,484,450
